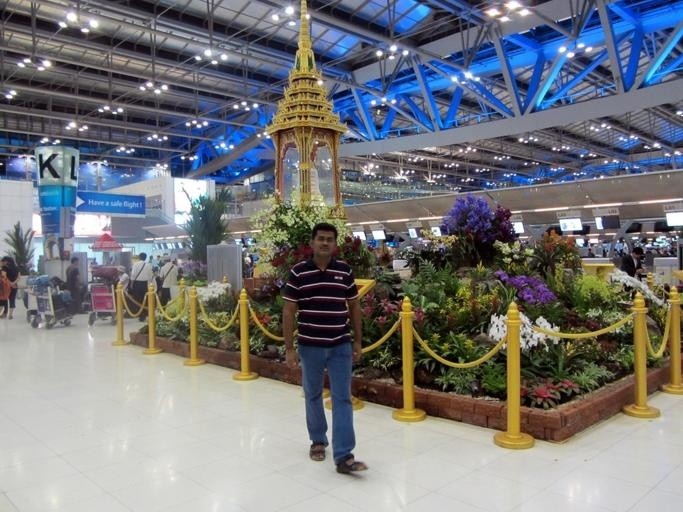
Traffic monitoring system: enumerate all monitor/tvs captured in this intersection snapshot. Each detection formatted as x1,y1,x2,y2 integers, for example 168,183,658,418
666,212,683,227
557,217,583,232
510,221,525,234
155,237,256,251
353,231,367,241
431,226,442,237
407,228,418,239
372,230,387,240
594,215,620,230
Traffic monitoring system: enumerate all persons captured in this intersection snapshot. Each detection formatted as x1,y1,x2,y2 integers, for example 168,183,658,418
583,237,663,299
277,222,369,474
541,226,563,239
0,252,193,323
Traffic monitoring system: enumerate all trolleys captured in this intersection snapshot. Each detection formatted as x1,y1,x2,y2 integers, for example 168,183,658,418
22,274,48,323
32,285,77,330
88,283,120,326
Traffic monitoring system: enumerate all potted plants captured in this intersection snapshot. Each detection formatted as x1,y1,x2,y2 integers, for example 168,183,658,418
0,220,36,288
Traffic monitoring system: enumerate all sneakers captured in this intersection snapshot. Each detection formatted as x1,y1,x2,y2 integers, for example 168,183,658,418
310,444,325,461
337,454,367,473
